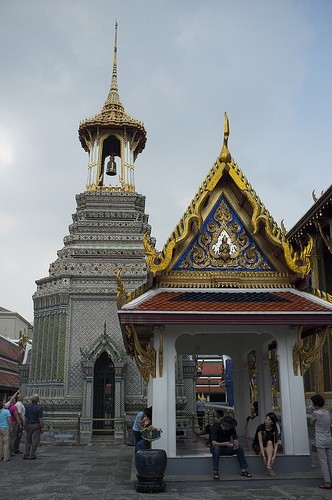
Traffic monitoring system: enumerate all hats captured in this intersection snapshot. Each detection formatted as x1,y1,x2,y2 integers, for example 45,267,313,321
220,415,237,428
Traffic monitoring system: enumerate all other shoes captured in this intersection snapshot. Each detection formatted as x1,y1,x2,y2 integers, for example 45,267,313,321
14,450,23,454
23,453,36,460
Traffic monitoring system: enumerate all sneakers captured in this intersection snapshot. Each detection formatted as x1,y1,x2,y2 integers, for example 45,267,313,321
319,482,332,488
241,470,252,477
212,472,220,479
265,468,277,477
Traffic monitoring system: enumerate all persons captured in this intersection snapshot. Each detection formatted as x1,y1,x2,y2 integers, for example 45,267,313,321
252,412,278,476
196,399,206,430
132,406,152,455
310,395,332,489
193,410,252,479
0,390,26,462
22,394,43,460
101,383,114,426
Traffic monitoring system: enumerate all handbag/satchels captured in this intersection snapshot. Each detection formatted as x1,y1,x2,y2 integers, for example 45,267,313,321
16,421,23,433
